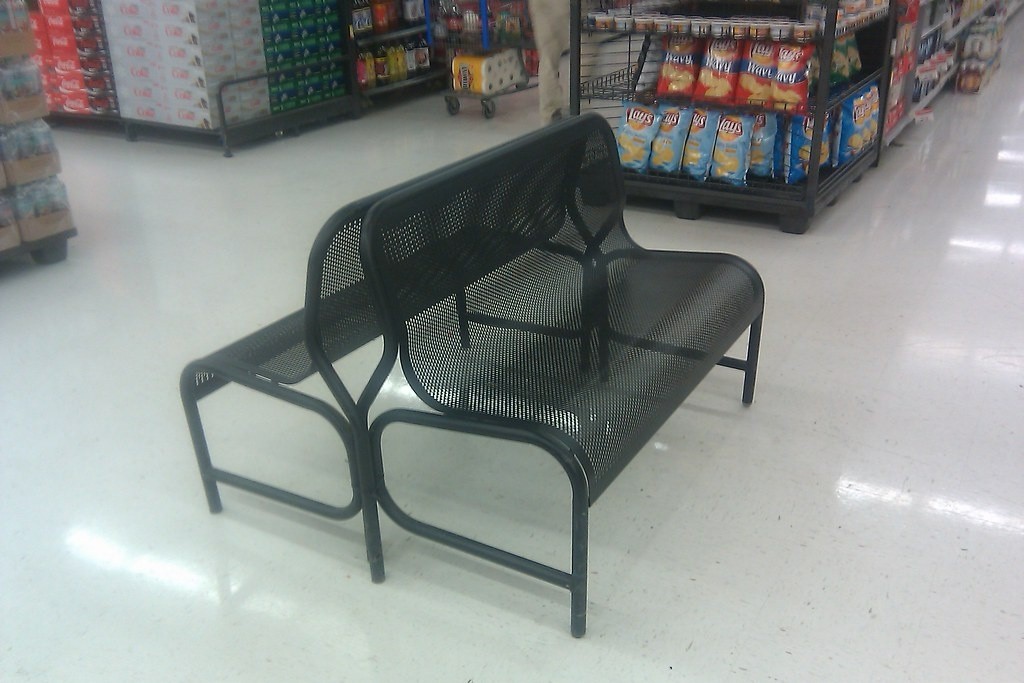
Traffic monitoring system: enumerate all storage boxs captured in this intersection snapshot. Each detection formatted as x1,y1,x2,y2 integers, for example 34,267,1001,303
0,0,345,251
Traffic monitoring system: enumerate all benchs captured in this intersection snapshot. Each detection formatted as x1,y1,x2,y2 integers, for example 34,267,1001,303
178,115,594,584
357,114,767,640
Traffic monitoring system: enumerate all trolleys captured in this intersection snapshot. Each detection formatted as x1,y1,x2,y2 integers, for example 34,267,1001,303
424,0,563,118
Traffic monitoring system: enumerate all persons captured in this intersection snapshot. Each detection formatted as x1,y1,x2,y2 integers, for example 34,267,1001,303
528,0,583,125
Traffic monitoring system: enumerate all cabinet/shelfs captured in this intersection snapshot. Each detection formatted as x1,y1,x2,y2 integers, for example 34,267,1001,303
358,21,446,109
568,1,1022,235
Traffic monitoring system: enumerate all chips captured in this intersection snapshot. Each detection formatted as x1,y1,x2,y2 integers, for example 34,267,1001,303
618,47,883,178
